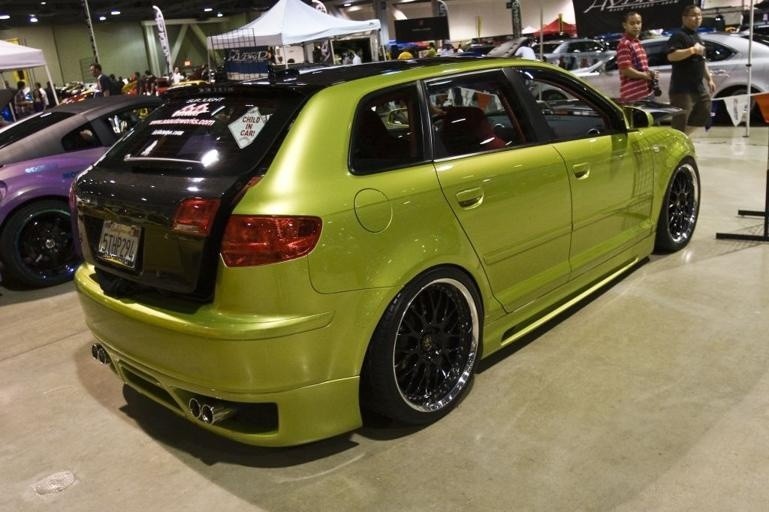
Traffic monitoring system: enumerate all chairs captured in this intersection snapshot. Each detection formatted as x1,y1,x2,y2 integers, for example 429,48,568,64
355,109,409,170
439,105,510,154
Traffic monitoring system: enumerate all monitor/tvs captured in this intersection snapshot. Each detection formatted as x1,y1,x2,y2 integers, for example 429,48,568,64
394,16,450,44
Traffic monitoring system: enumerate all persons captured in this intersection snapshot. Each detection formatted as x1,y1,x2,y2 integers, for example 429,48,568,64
665,4,717,127
615,9,660,100
513,41,538,60
